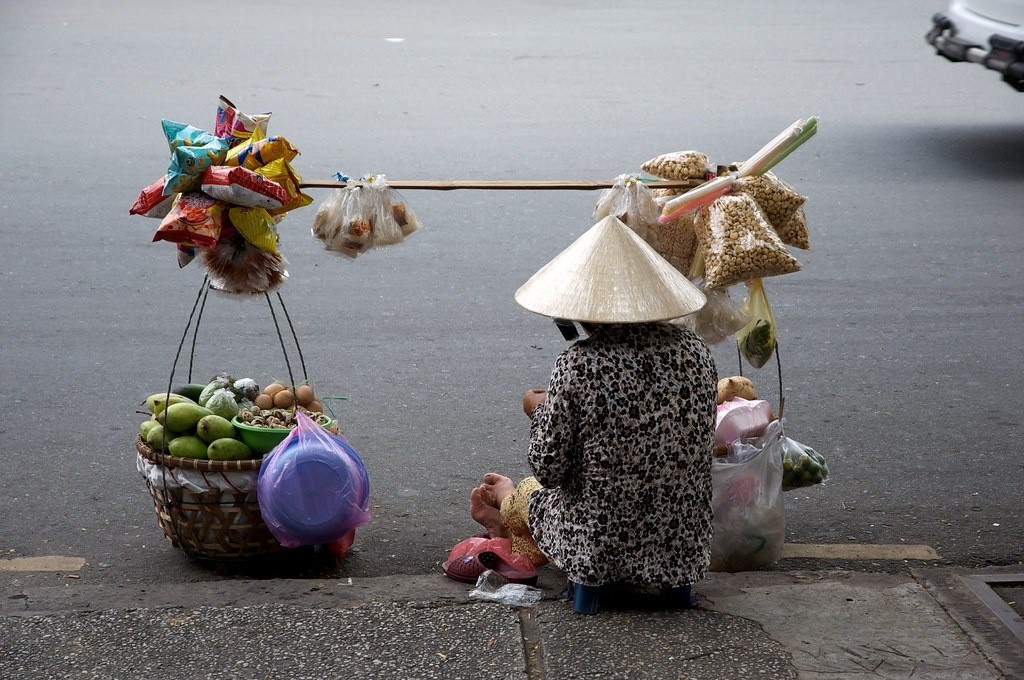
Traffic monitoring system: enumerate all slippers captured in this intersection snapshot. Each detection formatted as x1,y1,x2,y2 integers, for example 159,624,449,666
442,551,538,587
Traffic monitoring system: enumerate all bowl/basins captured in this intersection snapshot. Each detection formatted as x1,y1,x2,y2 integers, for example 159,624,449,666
714,399,770,446
231,411,332,453
258,435,369,545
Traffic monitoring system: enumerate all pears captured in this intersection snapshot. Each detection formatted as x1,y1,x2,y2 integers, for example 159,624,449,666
257,383,322,414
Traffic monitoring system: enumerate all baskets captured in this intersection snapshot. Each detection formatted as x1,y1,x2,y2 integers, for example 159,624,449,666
135,271,340,560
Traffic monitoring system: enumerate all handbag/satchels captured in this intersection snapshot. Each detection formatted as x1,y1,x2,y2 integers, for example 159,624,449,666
311,173,422,259
591,153,830,575
256,410,372,547
191,233,290,293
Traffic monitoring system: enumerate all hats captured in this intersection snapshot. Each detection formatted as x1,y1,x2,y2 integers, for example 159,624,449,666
515,215,707,323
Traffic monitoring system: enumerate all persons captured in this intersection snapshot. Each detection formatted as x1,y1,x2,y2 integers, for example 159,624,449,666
470,215,718,591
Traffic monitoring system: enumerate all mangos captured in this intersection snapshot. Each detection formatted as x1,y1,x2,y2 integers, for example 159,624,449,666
140,373,258,461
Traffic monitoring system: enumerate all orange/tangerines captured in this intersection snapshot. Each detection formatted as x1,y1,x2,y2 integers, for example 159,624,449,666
782,448,829,489
